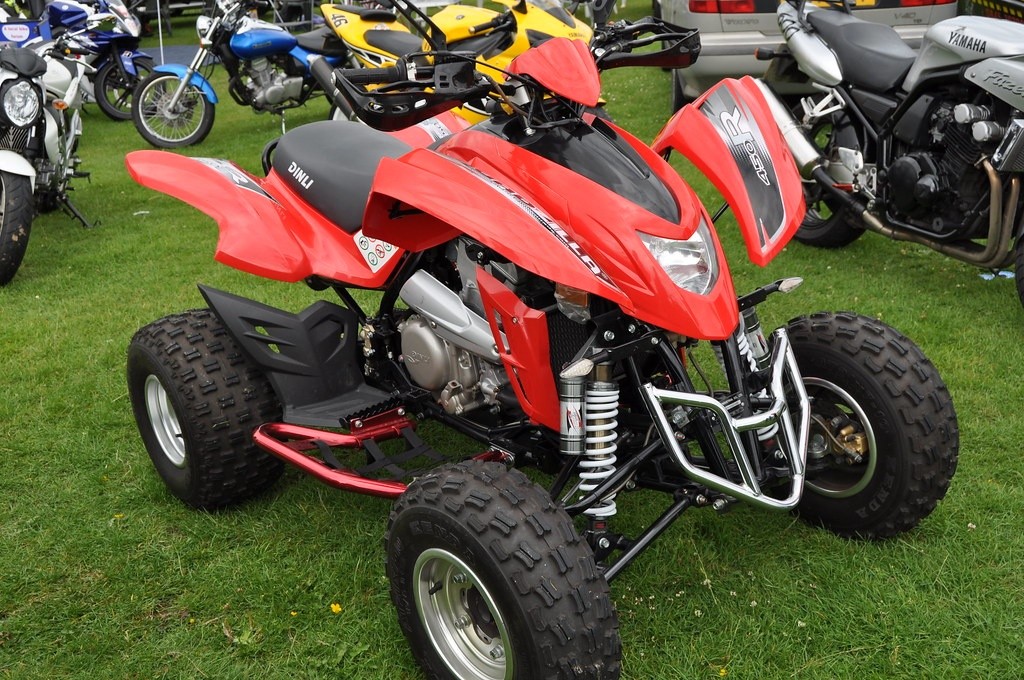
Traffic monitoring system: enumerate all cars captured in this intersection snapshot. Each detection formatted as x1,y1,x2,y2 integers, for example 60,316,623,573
650,0,962,117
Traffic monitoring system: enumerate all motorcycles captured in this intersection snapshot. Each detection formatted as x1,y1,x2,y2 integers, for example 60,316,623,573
752,1,1024,313
125,12,963,680
0,0,626,292
